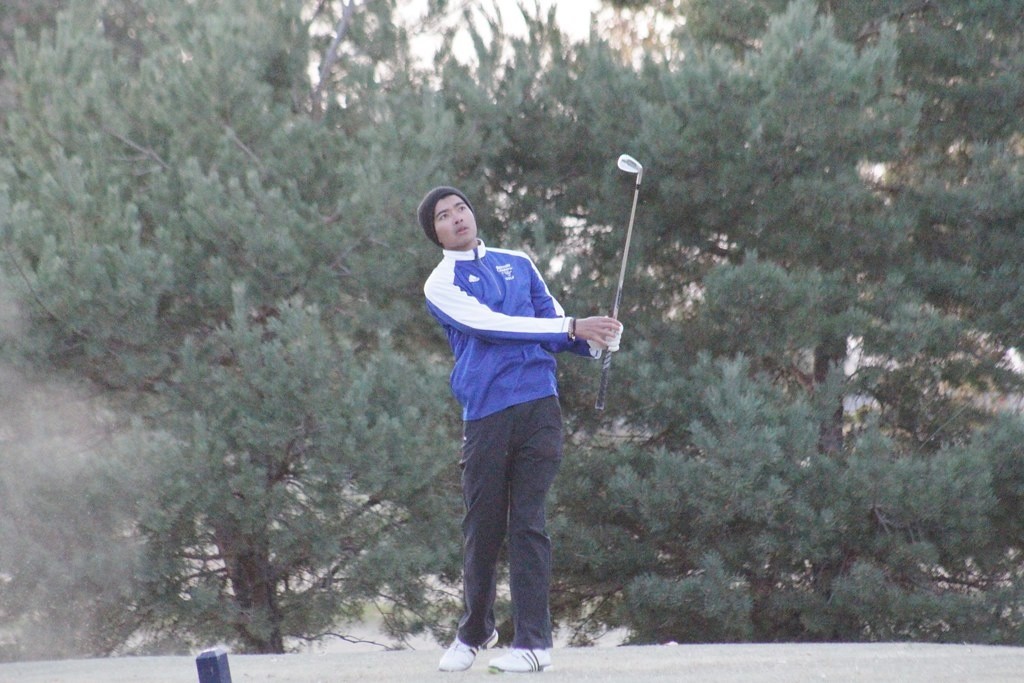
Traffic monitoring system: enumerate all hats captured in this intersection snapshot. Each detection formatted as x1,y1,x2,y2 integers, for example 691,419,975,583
417,186,474,248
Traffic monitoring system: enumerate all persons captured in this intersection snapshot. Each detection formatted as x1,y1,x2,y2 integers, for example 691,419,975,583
414,185,625,674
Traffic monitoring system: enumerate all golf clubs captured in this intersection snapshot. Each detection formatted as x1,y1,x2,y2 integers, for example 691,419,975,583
594,152,643,413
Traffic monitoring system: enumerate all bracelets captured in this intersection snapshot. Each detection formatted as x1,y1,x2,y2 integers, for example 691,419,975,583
568,319,572,340
571,318,577,341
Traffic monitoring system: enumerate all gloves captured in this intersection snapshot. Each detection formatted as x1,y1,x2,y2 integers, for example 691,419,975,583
586,314,624,353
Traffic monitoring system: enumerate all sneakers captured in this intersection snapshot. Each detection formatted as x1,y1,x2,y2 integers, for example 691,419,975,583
439,627,499,672
489,646,552,672
